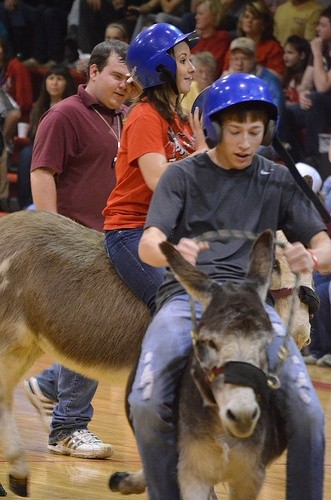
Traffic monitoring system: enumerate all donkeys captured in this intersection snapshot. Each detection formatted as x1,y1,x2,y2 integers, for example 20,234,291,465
160,228,285,499
0,208,311,495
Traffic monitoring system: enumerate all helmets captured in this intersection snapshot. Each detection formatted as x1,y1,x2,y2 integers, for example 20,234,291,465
126,23,201,90
201,73,281,149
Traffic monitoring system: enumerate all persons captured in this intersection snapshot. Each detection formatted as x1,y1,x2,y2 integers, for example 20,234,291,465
22,38,132,458
124,75,331,500
104,23,207,312
0,0,331,366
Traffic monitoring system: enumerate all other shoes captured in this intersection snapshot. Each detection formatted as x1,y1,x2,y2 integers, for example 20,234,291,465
302,353,331,367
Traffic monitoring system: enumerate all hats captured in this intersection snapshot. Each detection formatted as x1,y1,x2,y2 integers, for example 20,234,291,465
230,37,256,57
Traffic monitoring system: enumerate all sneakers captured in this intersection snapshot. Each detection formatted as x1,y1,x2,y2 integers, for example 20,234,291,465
23,376,60,434
47,429,114,459
